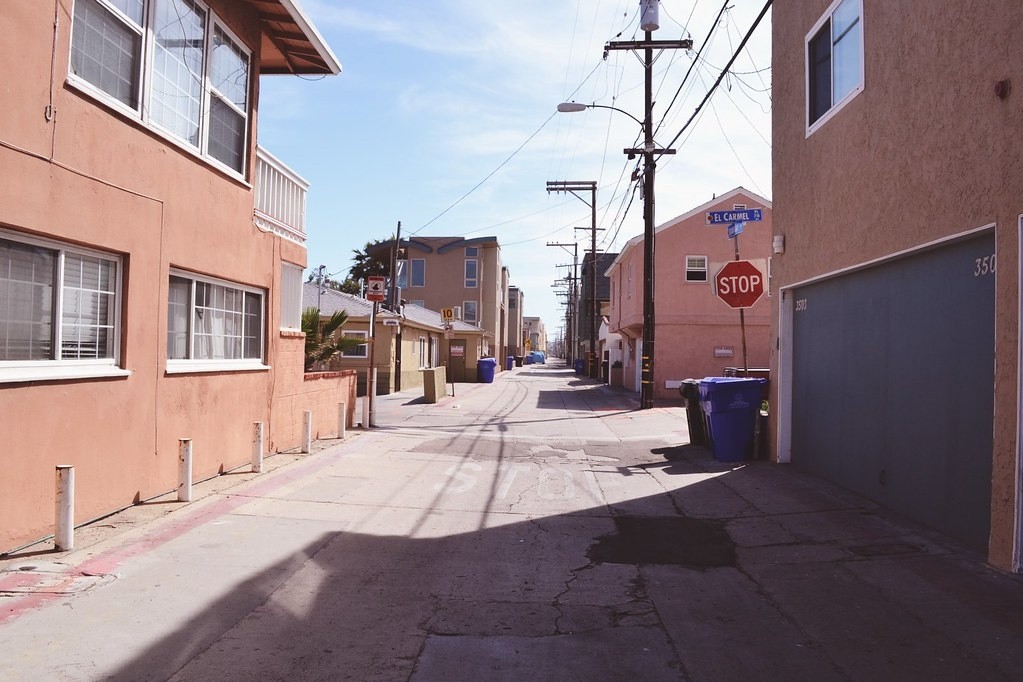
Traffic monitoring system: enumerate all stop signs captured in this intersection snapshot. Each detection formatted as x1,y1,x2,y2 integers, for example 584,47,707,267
715,260,765,309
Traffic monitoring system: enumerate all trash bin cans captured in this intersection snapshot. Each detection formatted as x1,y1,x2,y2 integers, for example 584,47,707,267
699,377,767,463
477,359,496,383
678,379,705,446
506,355,514,370
526,356,534,364
575,359,584,375
516,356,524,367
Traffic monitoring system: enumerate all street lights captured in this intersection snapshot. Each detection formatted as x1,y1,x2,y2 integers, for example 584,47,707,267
556,102,655,410
